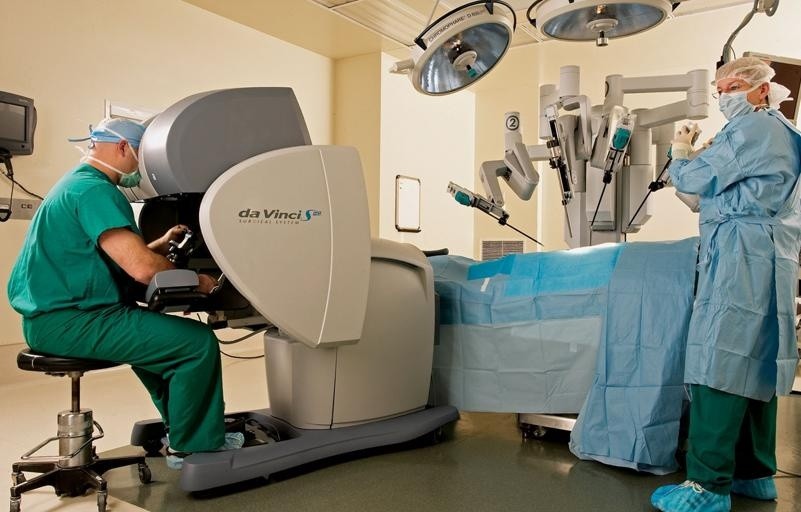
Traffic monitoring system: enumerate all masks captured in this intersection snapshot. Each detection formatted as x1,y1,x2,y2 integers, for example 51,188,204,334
118,151,141,188
718,87,766,121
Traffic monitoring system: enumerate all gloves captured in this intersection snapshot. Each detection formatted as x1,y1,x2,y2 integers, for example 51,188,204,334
670,122,714,160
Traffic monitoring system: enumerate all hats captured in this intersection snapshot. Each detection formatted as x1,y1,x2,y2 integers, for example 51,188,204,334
91,118,144,147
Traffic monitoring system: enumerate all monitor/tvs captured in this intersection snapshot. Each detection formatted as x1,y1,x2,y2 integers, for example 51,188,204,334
0,91,37,155
743,52,801,126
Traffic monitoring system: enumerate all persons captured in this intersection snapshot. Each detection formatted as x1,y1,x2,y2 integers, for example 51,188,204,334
5,114,247,468
649,55,801,512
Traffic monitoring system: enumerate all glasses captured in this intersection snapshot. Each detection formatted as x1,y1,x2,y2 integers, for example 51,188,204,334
712,82,746,100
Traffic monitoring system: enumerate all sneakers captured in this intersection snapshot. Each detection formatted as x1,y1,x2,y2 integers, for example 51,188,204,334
164,431,244,470
650,480,732,510
731,474,776,500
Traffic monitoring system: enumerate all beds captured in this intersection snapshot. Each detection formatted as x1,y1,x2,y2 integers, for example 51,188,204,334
418,231,703,467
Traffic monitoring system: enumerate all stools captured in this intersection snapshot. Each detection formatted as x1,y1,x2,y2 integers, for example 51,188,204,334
7,348,154,512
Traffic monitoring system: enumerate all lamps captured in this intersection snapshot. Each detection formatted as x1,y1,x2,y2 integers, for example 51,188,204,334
383,0,677,98
